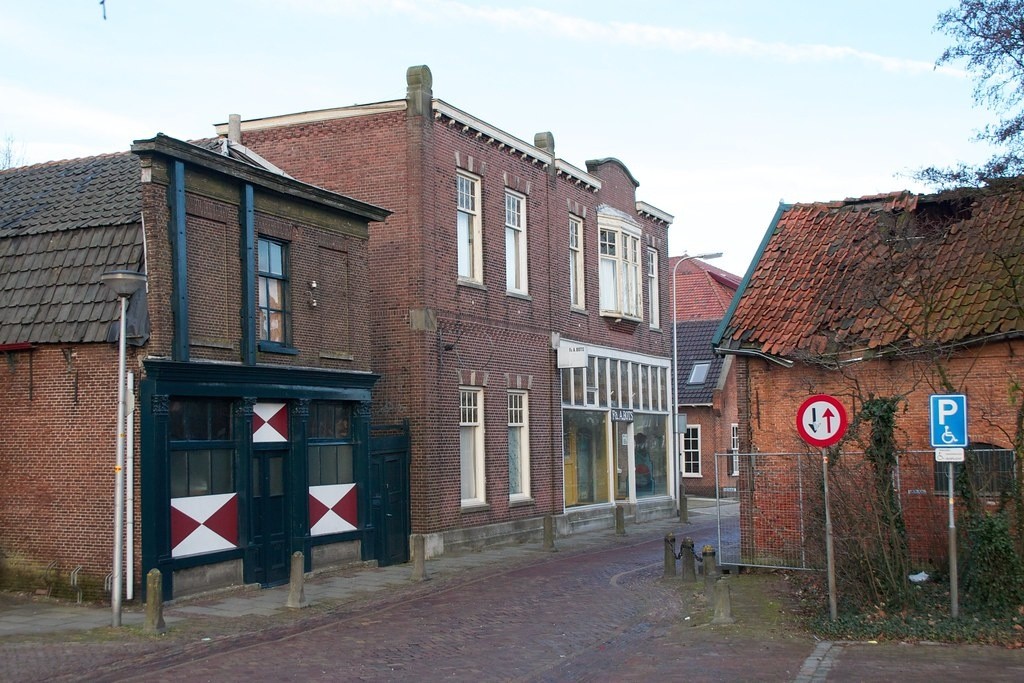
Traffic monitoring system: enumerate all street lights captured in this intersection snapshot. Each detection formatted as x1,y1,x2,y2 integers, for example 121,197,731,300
670,250,725,519
97,267,149,628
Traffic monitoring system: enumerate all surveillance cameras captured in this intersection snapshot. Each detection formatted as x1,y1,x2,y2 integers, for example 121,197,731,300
312,284,316,289
313,303,316,307
